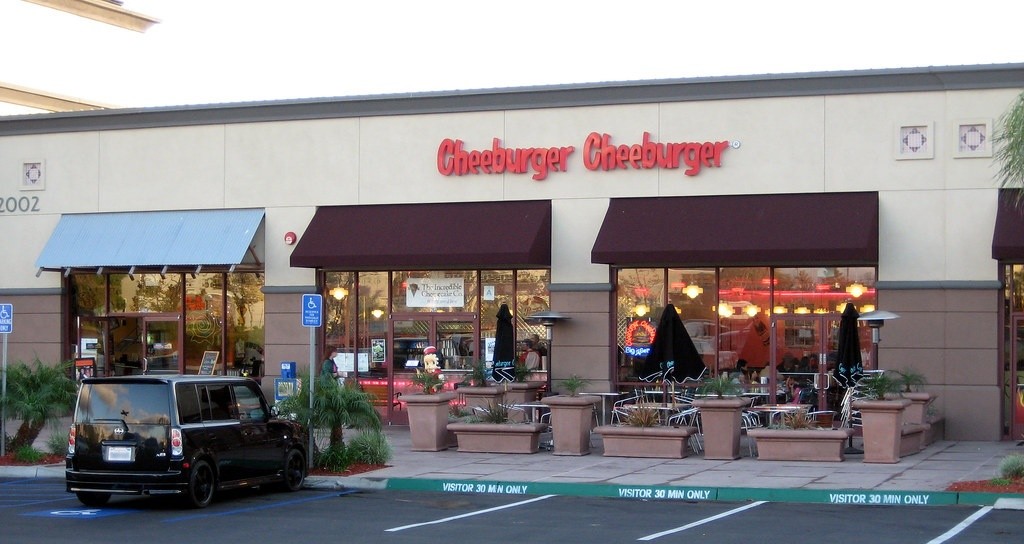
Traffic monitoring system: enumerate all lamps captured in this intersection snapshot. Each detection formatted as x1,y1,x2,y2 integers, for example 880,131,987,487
711,299,736,318
857,310,901,369
681,282,704,299
836,300,856,314
859,304,876,312
793,280,811,314
813,291,832,314
675,307,682,314
742,301,762,316
764,302,789,318
328,278,349,301
632,303,651,316
845,280,868,298
371,308,386,318
523,310,572,423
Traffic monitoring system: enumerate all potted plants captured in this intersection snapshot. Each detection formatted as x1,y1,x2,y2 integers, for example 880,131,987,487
444,396,549,453
395,363,457,457
456,357,546,421
693,370,752,459
593,400,699,457
540,374,602,457
849,364,945,464
747,404,857,463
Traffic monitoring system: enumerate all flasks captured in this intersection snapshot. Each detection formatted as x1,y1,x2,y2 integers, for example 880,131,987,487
542,356,547,370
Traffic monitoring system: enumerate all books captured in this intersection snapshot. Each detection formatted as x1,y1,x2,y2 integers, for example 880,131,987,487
412,341,458,366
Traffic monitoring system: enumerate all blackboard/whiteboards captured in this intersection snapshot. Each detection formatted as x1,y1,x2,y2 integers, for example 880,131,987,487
198,351,220,375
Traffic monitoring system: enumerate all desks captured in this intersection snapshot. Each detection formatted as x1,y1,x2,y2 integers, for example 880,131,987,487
578,391,632,426
744,392,780,425
694,391,748,397
631,402,692,425
509,400,549,423
644,389,681,402
753,404,811,425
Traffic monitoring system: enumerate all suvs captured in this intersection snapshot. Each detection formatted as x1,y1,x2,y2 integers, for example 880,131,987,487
370,338,469,376
65,373,319,508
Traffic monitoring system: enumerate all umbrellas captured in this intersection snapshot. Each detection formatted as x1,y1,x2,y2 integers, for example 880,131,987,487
491,305,517,392
831,302,864,449
638,304,707,416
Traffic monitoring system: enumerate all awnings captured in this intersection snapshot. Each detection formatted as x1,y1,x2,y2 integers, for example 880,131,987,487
288,201,552,268
590,192,877,263
990,189,1024,258
32,209,266,280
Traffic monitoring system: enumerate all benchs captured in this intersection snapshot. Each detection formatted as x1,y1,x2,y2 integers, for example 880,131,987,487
360,383,404,413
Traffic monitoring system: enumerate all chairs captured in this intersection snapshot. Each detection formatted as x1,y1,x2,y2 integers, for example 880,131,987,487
466,361,891,455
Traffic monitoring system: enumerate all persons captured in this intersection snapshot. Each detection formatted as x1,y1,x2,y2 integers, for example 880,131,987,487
319,344,340,390
778,351,819,384
518,335,547,370
78,366,87,380
416,345,444,392
727,358,802,404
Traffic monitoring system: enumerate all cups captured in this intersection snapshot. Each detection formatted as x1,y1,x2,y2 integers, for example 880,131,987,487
760,377,767,384
